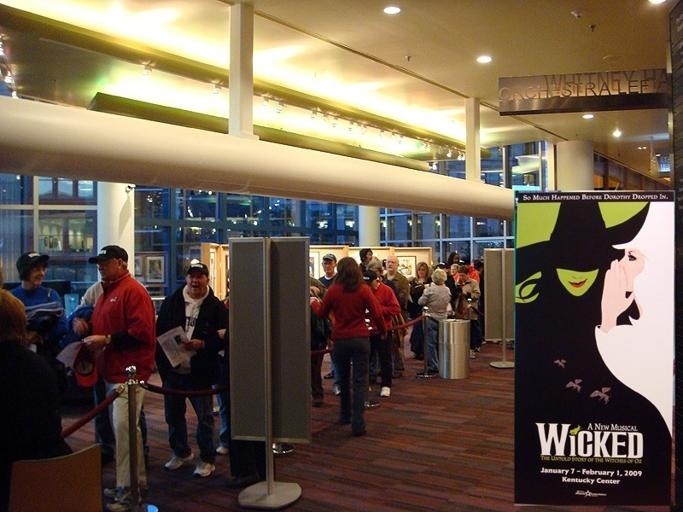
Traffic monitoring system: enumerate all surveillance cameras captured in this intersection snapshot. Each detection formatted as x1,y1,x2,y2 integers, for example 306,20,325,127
126,183,136,192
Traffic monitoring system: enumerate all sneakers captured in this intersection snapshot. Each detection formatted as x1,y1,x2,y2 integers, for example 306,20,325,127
165,454,193,470
104,486,144,512
380,387,391,397
193,461,216,478
417,371,437,378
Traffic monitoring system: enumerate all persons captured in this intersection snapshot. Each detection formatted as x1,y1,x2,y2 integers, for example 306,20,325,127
154,264,226,479
309,250,483,434
0,287,74,512
72,280,149,466
210,268,231,455
74,246,157,511
9,251,69,363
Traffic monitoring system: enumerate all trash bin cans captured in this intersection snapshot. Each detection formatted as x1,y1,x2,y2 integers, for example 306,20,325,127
438,318,471,380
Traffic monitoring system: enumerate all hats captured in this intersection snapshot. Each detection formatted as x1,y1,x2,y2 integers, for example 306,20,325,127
17,252,49,275
323,253,335,261
88,246,127,263
70,341,99,387
187,263,208,275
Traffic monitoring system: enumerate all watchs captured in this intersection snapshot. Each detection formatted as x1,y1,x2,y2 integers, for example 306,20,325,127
105,334,110,346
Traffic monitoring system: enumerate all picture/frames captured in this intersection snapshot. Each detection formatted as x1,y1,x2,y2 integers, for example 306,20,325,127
134,276,145,285
308,252,319,280
209,252,216,294
397,255,417,279
145,254,165,284
134,254,144,277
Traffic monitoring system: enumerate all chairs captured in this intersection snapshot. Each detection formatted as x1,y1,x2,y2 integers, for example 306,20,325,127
8,443,103,511
